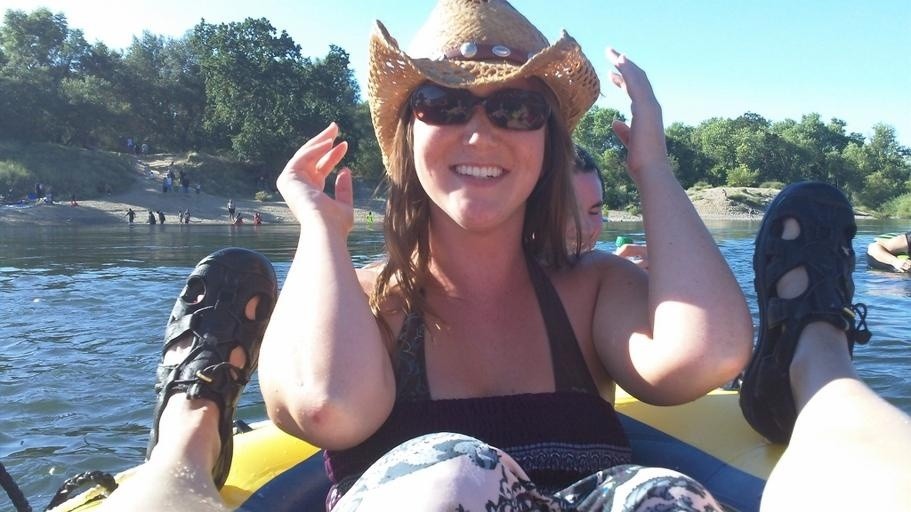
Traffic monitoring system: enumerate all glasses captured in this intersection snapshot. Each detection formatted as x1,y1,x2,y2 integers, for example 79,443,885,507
411,85,556,129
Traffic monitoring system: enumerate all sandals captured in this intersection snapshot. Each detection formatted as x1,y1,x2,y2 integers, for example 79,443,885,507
739,181,873,445
145,246,278,491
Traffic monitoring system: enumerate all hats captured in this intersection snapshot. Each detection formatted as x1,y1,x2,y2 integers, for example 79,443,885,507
367,0,601,190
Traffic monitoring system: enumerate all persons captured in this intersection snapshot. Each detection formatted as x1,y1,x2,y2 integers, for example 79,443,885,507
126,135,134,154
104,180,113,200
134,144,141,156
185,208,191,224
178,209,184,224
254,0,757,512
123,208,136,225
69,190,78,208
156,210,166,225
149,160,201,194
563,143,649,278
148,209,157,224
142,142,149,158
91,175,910,512
253,212,264,225
233,212,243,224
870,218,911,263
227,198,236,222
367,210,375,224
